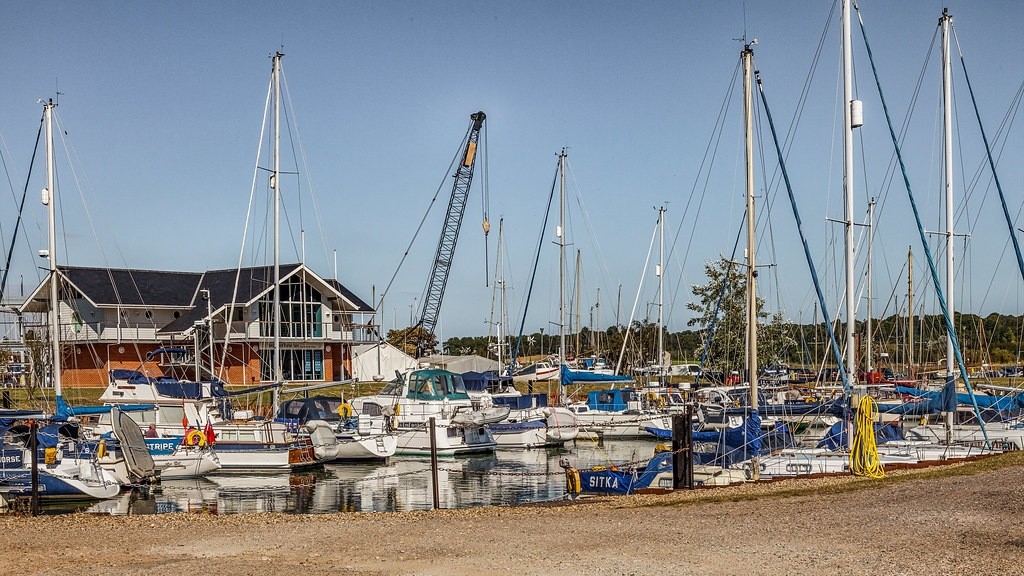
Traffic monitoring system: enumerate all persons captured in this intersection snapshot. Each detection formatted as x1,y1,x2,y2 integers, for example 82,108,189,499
146,423,159,438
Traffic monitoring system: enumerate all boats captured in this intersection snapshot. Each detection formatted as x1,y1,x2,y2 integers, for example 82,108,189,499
484,352,1024,508
95,366,398,478
568,420,1024,494
345,369,512,459
0,409,123,500
19,403,221,482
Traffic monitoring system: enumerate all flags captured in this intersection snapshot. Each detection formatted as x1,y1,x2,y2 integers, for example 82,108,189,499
204,423,217,447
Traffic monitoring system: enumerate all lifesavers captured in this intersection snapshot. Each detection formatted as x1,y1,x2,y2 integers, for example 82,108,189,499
338,403,352,417
187,430,206,446
644,392,666,408
98,439,108,458
